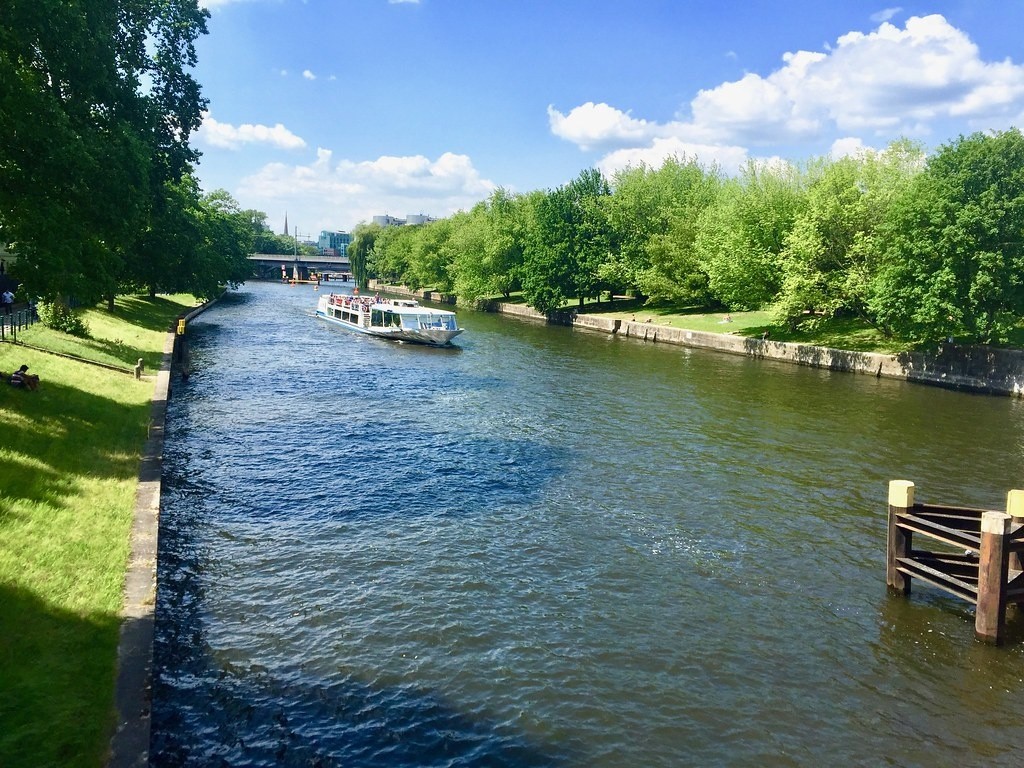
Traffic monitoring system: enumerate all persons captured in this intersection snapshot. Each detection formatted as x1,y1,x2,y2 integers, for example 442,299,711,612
11,365,40,393
2,288,16,314
631,314,636,321
761,329,771,339
374,292,380,303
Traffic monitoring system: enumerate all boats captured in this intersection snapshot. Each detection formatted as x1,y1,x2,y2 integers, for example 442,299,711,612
315,292,466,347
